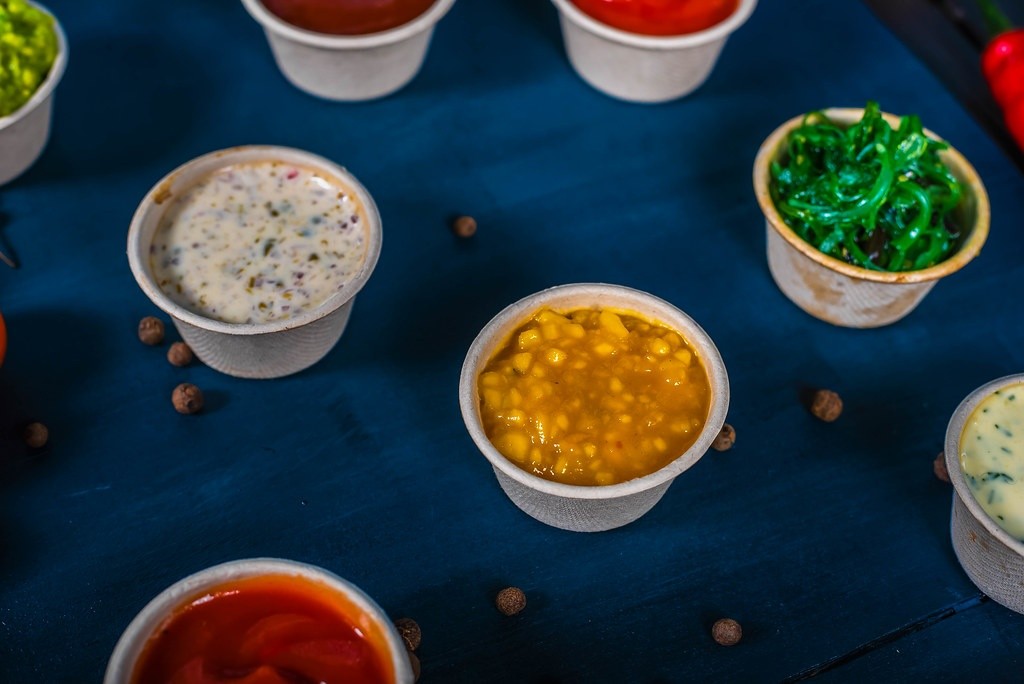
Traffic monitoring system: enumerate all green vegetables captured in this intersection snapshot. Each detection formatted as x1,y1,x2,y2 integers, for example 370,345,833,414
769,102,963,274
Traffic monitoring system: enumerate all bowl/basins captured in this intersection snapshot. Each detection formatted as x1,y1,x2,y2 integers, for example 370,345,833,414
548,0,758,104
103,559,417,684
945,373,1024,616
457,282,730,535
0,0,69,186
241,0,456,103
752,107,992,329
126,144,383,380
